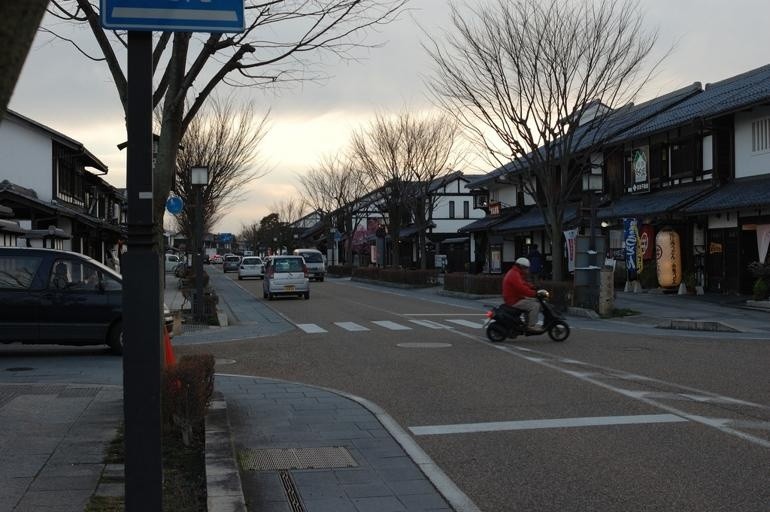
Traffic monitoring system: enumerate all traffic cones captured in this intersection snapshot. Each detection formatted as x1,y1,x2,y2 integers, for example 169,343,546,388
164,324,185,391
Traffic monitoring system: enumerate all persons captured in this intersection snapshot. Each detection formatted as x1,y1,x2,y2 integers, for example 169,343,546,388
525,244,542,283
54,264,67,292
502,258,544,332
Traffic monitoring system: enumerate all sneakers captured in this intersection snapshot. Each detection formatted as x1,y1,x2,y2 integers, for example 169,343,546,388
529,326,544,332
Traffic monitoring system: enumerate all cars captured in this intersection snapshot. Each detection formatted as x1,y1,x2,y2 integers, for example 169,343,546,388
207,252,269,273
235,255,265,281
164,253,182,272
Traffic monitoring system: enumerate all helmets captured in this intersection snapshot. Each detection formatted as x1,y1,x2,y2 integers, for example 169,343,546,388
515,258,531,268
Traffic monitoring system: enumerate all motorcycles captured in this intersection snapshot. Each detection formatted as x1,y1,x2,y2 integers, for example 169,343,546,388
480,286,572,343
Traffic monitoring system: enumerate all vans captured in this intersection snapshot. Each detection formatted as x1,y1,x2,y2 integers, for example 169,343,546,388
261,254,311,300
293,247,326,283
0,243,181,353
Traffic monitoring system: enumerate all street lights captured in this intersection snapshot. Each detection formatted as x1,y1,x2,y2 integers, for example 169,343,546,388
188,164,212,322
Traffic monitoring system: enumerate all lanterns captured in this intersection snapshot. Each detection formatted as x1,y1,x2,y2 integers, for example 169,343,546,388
656,227,682,287
